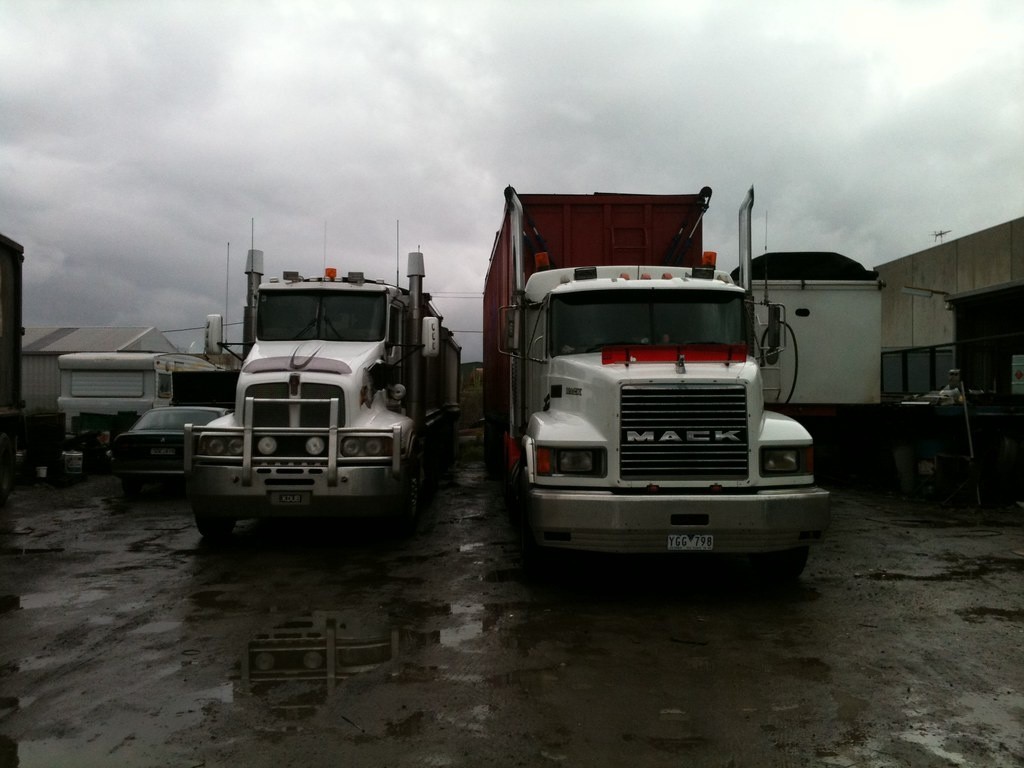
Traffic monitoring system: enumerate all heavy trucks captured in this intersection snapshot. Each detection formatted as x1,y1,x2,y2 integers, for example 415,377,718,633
481,185,832,588
0,233,26,504
182,247,464,547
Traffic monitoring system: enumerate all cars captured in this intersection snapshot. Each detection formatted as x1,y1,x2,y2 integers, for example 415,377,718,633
106,406,234,501
